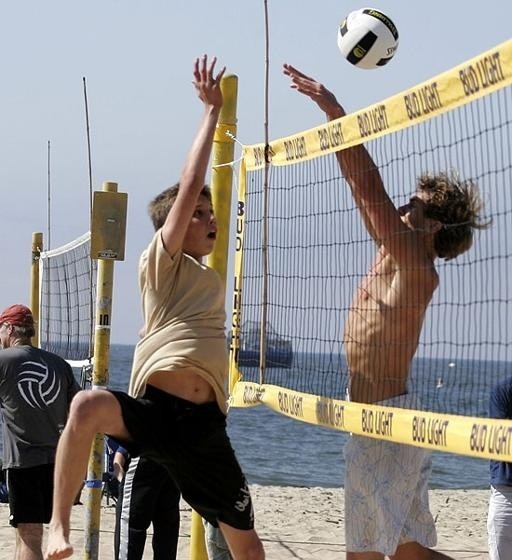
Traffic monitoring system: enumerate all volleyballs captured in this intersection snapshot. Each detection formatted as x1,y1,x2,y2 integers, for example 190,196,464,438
337,7,399,70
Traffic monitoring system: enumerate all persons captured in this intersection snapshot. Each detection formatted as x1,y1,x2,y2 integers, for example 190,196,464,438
1,304,81,559
110,441,182,560
39,51,268,559
281,58,488,560
484,373,511,560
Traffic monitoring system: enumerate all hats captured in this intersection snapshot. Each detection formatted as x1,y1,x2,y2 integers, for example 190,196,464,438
0,303,34,327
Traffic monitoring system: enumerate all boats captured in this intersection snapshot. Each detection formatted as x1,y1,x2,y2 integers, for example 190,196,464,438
227,319,293,369
